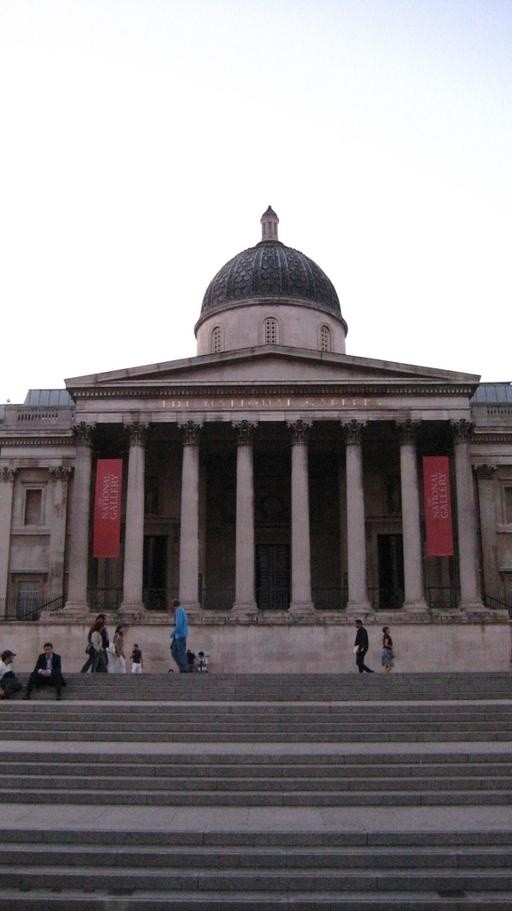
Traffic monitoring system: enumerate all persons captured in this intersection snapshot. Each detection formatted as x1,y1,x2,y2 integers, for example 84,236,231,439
170,599,208,673
354,620,375,673
0,651,21,699
22,643,66,700
80,614,126,673
130,643,143,673
381,626,396,672
